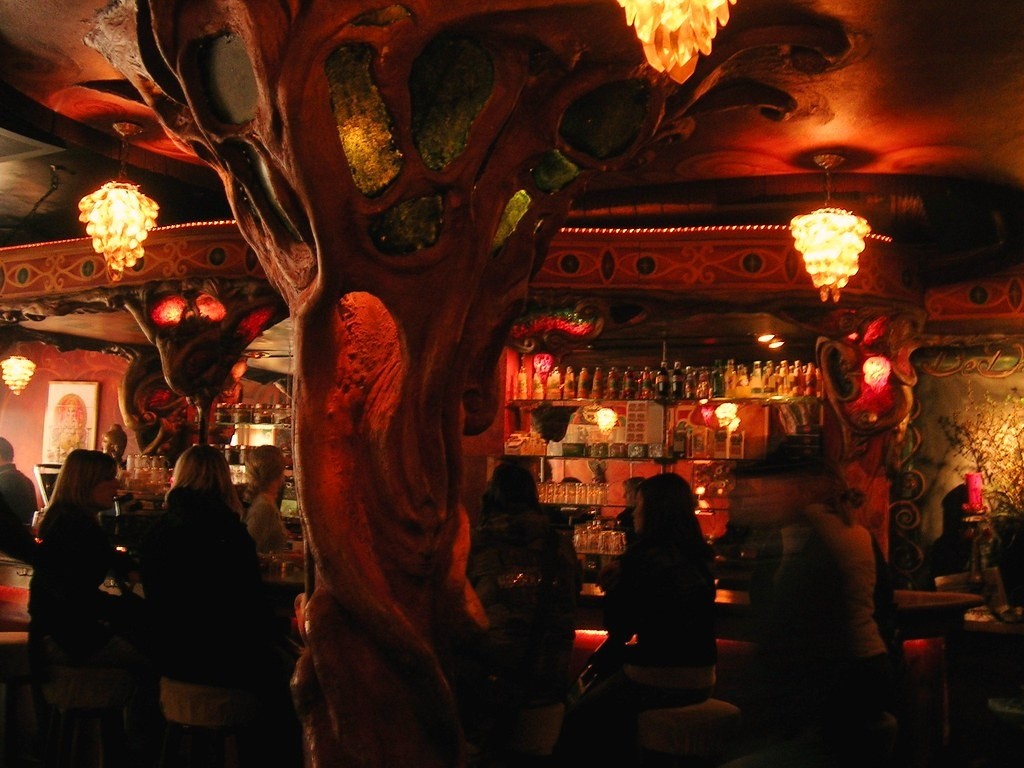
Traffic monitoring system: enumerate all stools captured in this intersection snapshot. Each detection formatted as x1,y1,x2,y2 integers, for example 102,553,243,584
0,629,28,768
853,714,898,764
154,679,242,768
26,665,128,767
505,703,569,757
640,697,741,768
987,695,1024,731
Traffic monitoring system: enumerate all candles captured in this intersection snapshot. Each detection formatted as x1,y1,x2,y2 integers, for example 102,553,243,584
960,472,985,512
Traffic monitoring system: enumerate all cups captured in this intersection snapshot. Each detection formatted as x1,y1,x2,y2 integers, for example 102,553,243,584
572,525,627,553
535,481,611,505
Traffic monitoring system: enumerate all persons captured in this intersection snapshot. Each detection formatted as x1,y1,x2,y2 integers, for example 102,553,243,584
554,471,719,768
0,435,40,524
919,484,1024,767
140,445,306,768
20,449,142,668
472,462,585,712
801,486,890,765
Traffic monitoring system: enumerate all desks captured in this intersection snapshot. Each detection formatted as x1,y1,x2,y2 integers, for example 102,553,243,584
966,604,1024,635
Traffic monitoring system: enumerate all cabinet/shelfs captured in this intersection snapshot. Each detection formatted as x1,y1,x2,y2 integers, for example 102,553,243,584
504,394,830,558
113,420,302,531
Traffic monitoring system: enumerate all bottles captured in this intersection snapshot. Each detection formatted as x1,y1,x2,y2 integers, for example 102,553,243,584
224,445,237,464
544,366,655,399
517,367,528,401
216,403,232,422
239,444,249,462
126,455,174,488
672,421,746,460
274,402,291,424
657,359,823,400
234,402,251,424
254,403,272,424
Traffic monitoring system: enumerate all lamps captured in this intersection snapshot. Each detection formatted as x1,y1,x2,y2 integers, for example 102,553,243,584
0,344,37,398
789,152,871,302
617,0,738,85
594,401,618,436
78,122,160,281
715,402,744,435
